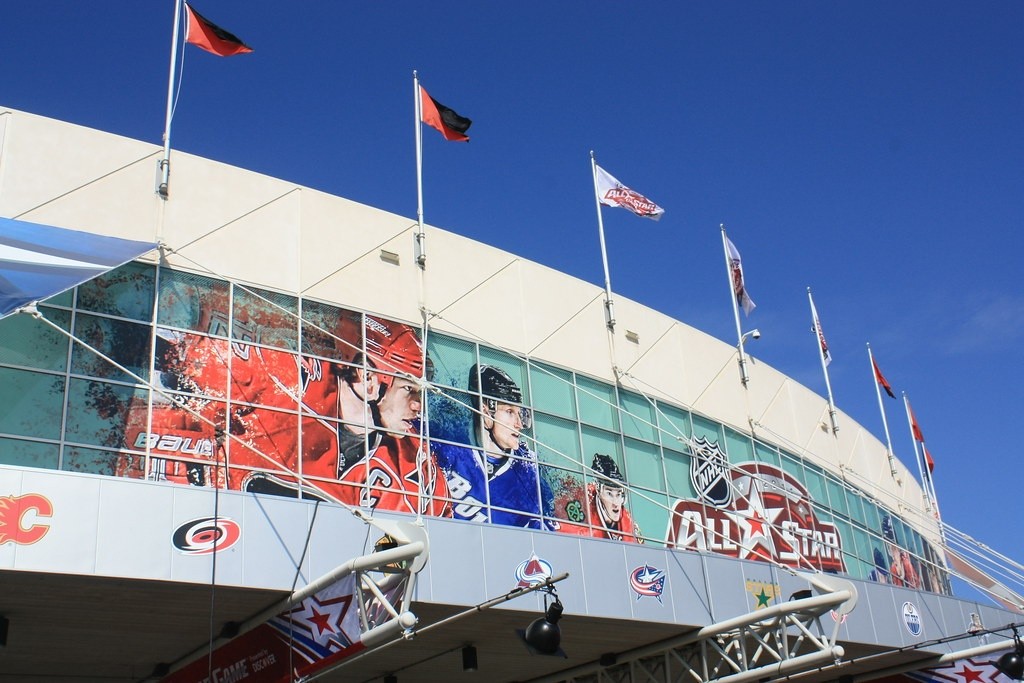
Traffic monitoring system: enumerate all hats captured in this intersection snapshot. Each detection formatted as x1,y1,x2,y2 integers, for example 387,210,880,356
874,548,889,575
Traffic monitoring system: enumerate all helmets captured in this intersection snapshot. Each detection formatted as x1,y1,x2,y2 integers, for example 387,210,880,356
590,453,628,504
334,310,435,387
881,516,898,544
468,363,523,417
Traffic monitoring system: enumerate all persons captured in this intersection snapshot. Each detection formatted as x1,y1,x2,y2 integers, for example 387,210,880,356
414,362,554,533
548,454,643,544
123,277,452,518
867,516,920,589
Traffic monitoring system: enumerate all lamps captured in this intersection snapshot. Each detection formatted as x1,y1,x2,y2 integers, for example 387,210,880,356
988,630,1024,680
461,647,477,673
514,587,567,658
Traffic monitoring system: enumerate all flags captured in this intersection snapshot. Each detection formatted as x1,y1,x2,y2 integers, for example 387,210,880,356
871,360,895,399
417,88,472,143
812,301,832,368
907,404,923,441
726,239,757,316
922,448,934,481
183,3,253,57
595,167,664,223
